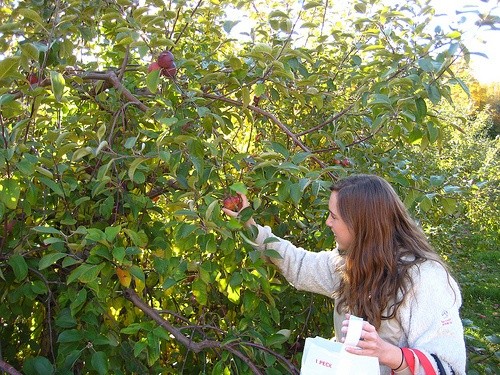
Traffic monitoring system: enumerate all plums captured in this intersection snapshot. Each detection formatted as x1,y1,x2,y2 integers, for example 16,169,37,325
225,196,243,212
27,74,40,85
333,159,349,167
147,51,177,78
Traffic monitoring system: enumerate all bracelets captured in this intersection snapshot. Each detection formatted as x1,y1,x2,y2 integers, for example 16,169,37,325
391,347,405,371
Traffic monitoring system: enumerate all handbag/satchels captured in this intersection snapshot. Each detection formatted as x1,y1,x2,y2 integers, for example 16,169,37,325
299,315,382,375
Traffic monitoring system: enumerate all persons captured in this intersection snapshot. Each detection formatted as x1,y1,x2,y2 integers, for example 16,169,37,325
221,173,468,375
